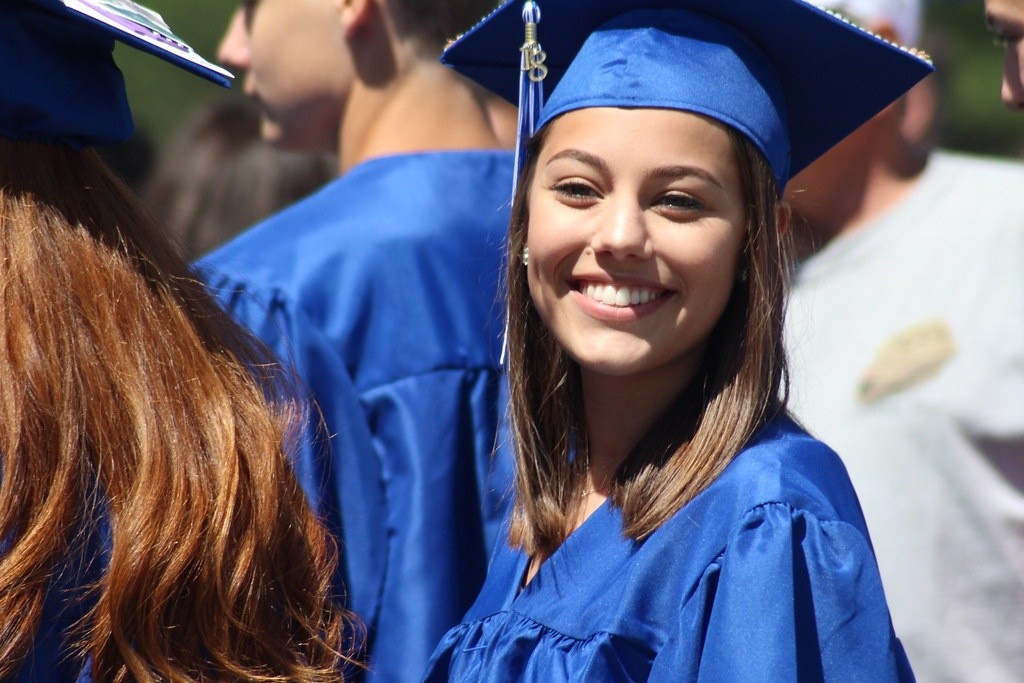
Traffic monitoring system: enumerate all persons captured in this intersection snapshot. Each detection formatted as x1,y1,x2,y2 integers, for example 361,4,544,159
0,0,1024,683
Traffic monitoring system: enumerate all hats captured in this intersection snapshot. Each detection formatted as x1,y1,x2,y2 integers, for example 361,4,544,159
438,0,935,366
0,0,235,149
